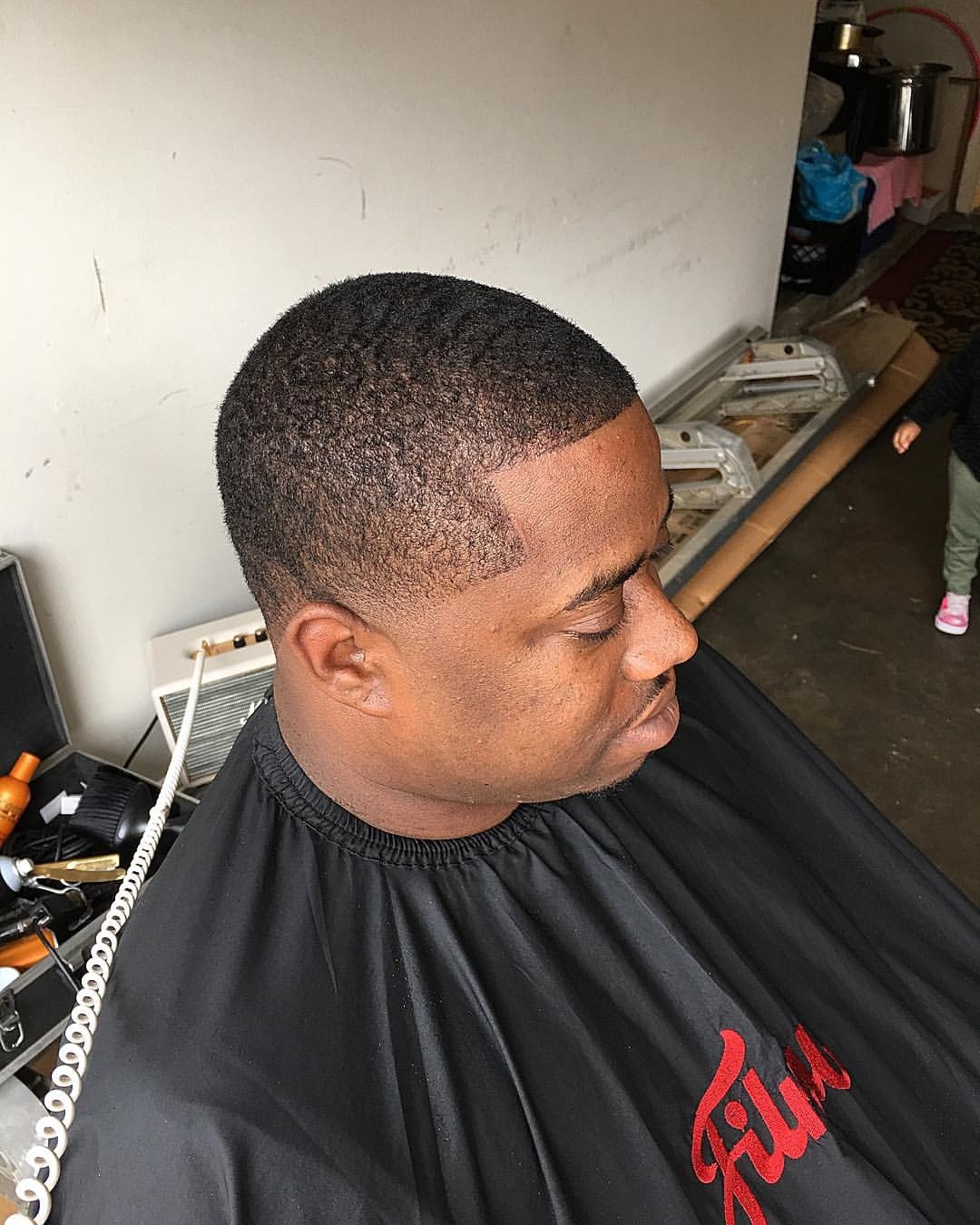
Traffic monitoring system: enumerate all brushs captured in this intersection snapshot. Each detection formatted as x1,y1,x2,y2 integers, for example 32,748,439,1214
65,762,195,847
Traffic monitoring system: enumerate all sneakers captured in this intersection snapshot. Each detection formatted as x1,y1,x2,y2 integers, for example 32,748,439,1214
935,594,973,636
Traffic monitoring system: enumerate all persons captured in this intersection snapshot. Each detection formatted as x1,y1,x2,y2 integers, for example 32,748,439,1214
892,331,980,636
25,269,976,1220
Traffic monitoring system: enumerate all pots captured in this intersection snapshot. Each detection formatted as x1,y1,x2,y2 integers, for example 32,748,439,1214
812,23,885,135
864,62,951,156
